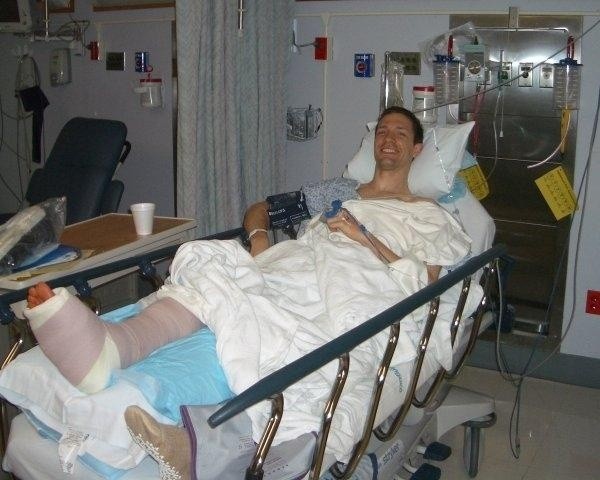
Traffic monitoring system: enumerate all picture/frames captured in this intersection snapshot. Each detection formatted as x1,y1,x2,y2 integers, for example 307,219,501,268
2,211,200,311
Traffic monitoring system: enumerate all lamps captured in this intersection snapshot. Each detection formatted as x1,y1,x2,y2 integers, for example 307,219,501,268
24,118,132,221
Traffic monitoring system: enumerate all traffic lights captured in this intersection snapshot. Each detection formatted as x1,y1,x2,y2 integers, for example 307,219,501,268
350,119,479,199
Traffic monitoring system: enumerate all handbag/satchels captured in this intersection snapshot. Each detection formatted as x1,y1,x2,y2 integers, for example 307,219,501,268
247,229,268,239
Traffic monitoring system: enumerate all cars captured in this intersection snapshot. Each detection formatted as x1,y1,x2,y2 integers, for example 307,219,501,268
130,202,156,235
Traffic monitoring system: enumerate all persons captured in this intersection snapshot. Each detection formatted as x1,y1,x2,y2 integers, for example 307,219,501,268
26,106,448,480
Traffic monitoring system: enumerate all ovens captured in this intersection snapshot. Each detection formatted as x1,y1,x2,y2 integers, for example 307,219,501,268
2,122,512,480
